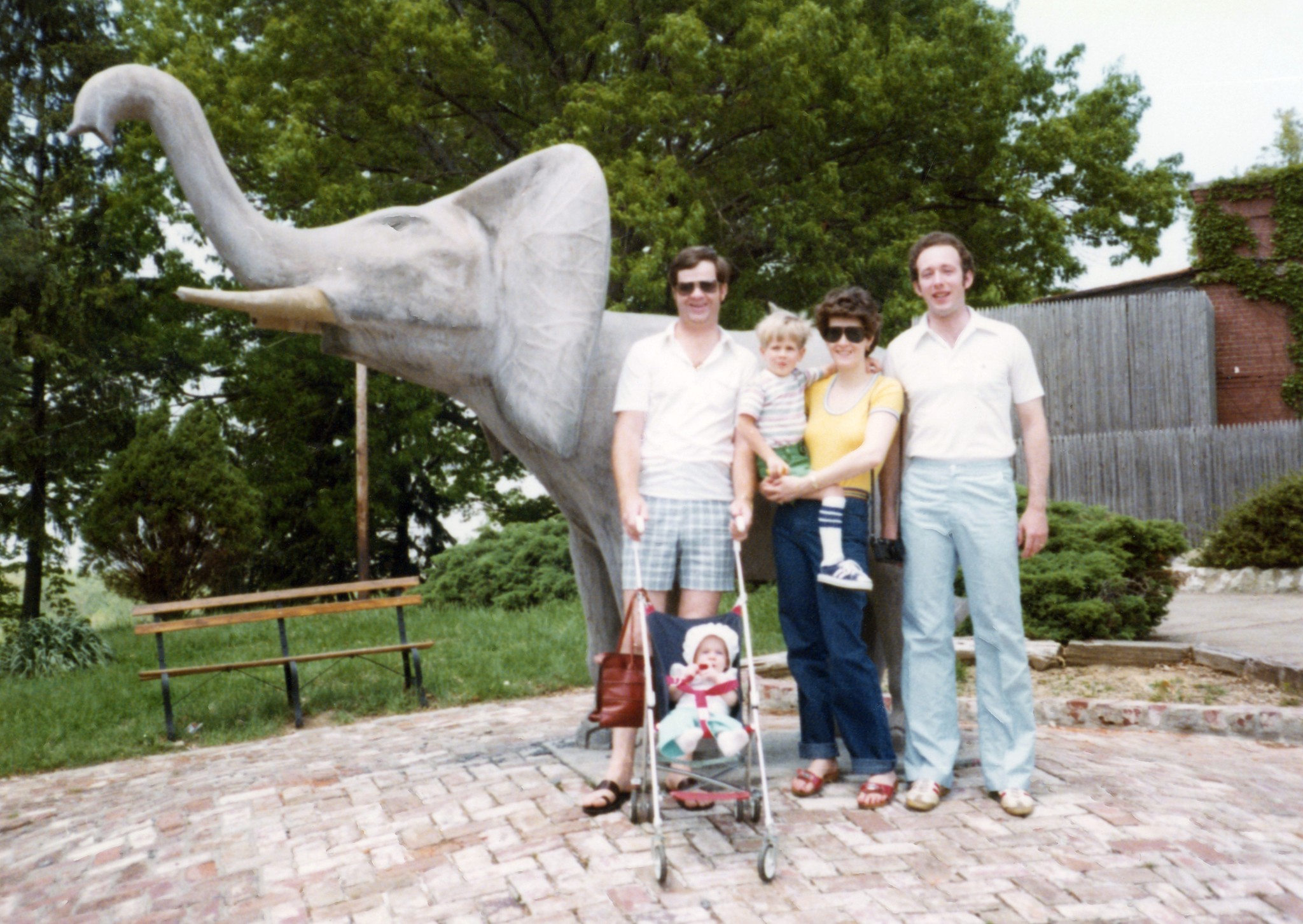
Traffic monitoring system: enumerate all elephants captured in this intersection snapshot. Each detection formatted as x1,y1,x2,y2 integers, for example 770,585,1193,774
67,49,956,802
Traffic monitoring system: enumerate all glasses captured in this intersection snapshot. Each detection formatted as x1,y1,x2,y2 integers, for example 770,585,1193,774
822,324,867,344
676,280,716,296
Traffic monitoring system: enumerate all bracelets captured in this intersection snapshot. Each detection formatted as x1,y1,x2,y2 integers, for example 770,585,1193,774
809,476,819,491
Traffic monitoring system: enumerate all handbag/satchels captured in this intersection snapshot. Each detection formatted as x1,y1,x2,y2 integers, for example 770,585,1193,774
587,587,658,729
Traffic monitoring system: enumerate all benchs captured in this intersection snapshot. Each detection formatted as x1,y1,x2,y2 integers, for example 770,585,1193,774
130,577,433,742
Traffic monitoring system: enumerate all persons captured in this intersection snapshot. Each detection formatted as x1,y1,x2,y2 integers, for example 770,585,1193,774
735,301,886,590
760,287,905,811
575,245,755,820
884,230,1050,817
656,622,749,757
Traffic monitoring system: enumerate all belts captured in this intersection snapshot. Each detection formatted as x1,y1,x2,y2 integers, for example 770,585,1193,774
843,489,868,501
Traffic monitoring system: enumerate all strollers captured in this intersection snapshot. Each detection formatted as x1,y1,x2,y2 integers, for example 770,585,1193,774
628,514,779,882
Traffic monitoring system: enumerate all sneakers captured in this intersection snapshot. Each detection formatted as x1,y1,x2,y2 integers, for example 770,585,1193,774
815,558,873,593
997,790,1035,816
905,779,943,811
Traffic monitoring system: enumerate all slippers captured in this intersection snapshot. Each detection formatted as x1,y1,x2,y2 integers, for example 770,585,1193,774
857,774,898,808
665,777,714,811
582,780,632,815
790,767,839,797
